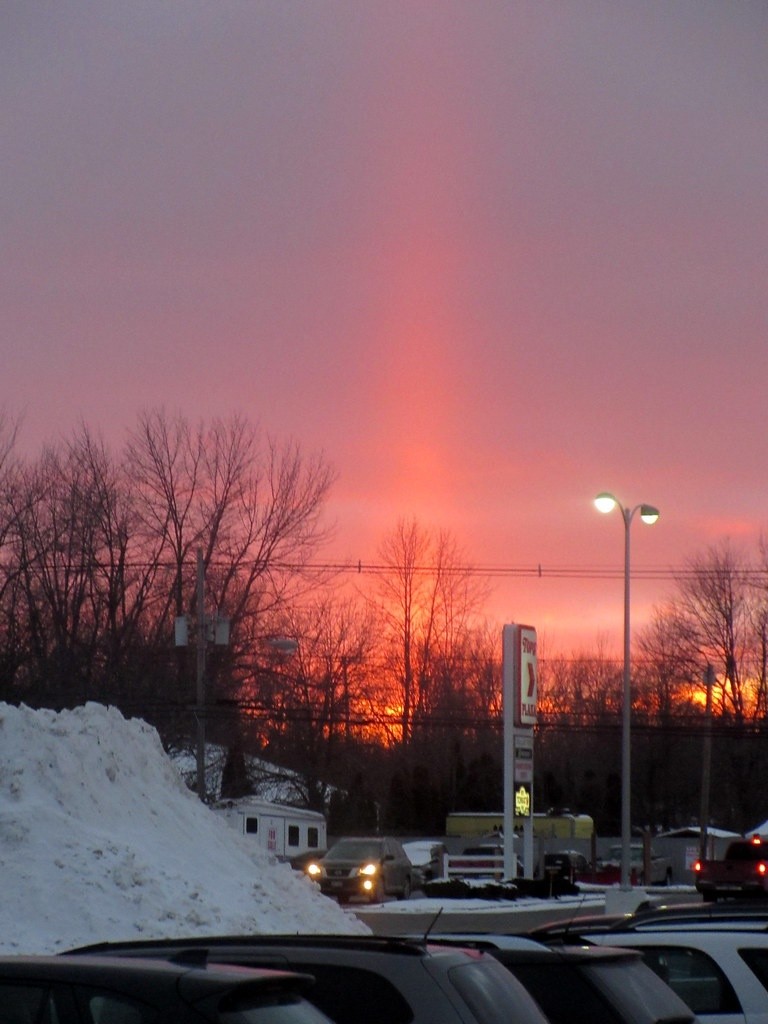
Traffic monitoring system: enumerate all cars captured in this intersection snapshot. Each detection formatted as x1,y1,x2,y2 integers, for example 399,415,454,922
461,843,524,880
0,948,330,1022
401,840,451,885
533,850,590,883
590,845,673,887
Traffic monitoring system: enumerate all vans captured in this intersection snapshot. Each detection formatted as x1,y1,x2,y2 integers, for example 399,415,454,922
308,837,413,905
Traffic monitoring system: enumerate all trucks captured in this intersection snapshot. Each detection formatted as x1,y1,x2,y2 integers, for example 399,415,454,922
694,836,768,904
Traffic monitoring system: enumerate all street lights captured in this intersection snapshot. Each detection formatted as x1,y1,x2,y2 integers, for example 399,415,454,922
594,491,661,890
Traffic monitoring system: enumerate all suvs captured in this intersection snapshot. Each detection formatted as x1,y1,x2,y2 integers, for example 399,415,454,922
56,934,546,1024
400,898,767,1024
